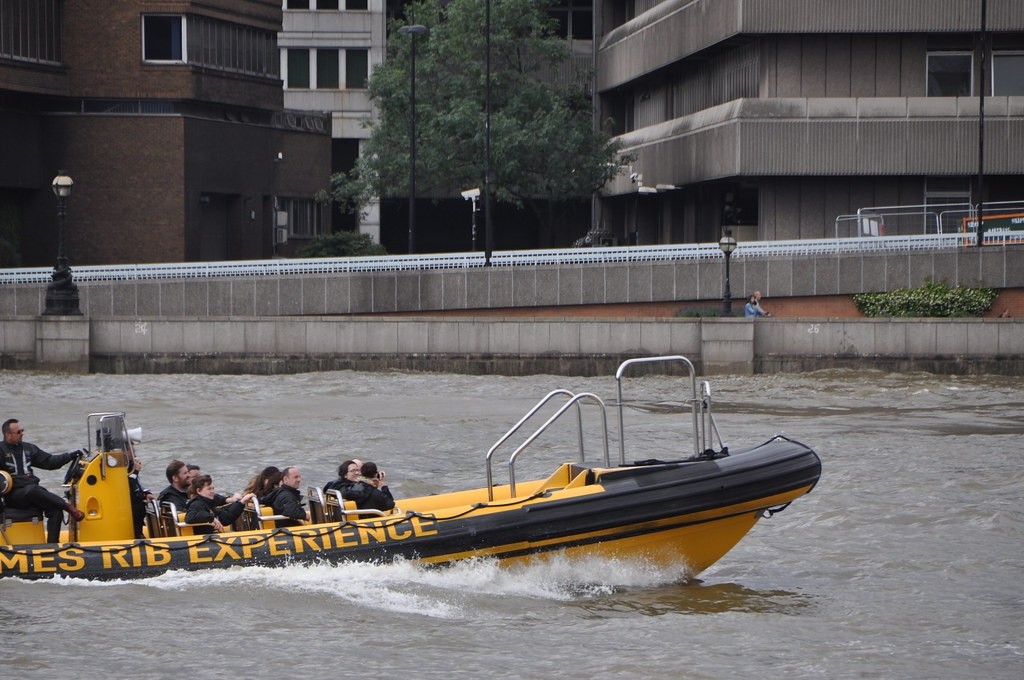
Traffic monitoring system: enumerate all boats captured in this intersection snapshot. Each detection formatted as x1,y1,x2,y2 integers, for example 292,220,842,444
0,356,825,604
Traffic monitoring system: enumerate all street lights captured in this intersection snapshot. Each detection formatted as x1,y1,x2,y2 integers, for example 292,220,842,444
396,24,430,253
720,227,738,316
40,171,87,317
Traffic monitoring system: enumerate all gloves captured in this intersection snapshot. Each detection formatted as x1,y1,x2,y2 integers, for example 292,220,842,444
69,448,90,459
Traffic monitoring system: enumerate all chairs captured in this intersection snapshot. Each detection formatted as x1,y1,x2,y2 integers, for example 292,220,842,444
307,485,387,525
227,492,291,532
144,499,225,538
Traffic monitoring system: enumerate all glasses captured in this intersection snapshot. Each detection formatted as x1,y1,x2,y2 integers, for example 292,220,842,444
9,429,24,434
347,469,359,473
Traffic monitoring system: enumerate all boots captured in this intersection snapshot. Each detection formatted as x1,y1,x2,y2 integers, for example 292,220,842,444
63,502,85,521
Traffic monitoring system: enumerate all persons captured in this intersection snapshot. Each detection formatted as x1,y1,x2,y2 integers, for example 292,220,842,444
244,466,310,528
324,457,395,519
745,290,771,319
156,460,256,533
0,418,85,543
126,441,154,539
